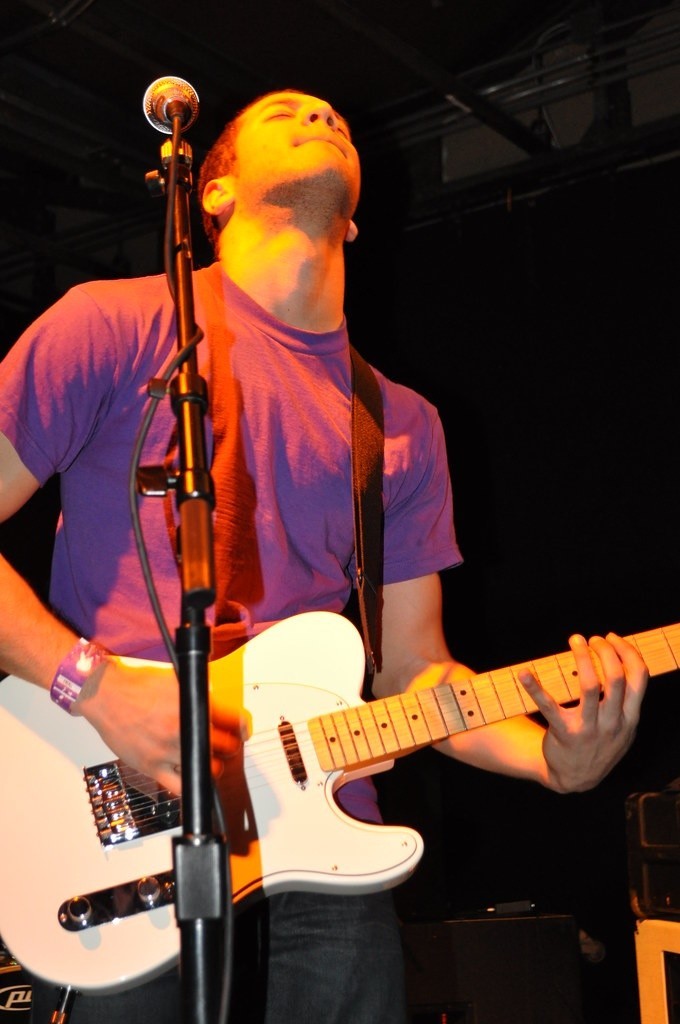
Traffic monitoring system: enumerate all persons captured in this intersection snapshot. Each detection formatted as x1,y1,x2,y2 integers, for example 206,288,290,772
0,90,648,1024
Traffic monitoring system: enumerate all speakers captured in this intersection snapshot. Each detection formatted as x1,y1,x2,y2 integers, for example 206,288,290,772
635,920,680,1024
403,917,578,1024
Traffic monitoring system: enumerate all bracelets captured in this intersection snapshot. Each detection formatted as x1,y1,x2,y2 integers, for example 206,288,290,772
50,638,110,715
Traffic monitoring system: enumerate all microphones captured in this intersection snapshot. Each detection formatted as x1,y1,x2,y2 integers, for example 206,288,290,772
143,77,200,135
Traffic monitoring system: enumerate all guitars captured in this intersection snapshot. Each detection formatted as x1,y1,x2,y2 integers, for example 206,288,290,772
1,605,678,996
